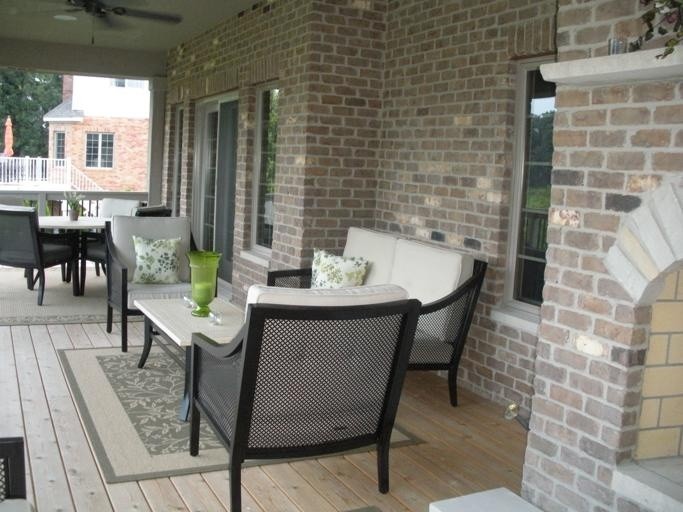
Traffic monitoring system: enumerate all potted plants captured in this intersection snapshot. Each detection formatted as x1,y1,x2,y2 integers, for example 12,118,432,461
63,189,84,221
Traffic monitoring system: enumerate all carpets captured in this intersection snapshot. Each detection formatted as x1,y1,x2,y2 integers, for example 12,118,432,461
51,344,426,484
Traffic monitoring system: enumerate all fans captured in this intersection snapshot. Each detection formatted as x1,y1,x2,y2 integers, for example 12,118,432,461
54,0,183,33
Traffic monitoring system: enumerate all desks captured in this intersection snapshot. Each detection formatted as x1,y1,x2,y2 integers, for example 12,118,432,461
37,214,106,293
136,296,244,420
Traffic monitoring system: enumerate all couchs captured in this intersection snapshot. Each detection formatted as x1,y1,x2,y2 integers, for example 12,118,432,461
268,226,488,407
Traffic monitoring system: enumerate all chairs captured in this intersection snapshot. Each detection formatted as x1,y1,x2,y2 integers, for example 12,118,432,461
186,281,423,508
132,202,172,215
2,202,79,303
102,215,201,353
